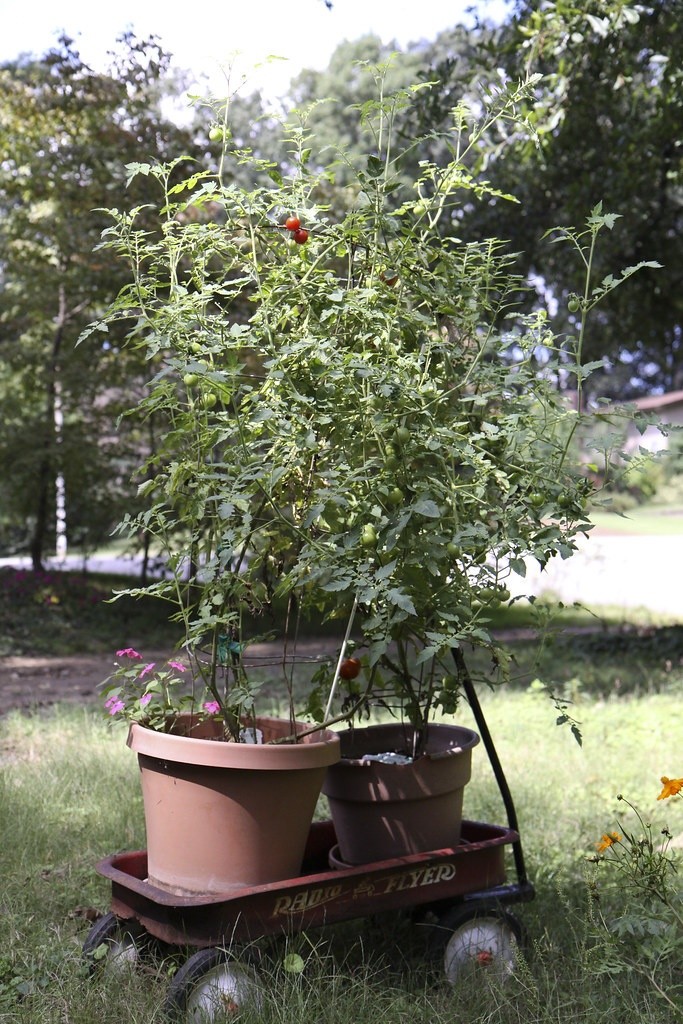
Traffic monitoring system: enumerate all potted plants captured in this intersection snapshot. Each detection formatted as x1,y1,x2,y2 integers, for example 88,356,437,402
72,49,663,897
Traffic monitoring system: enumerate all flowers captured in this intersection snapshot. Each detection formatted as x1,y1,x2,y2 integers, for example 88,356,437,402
94,648,221,735
